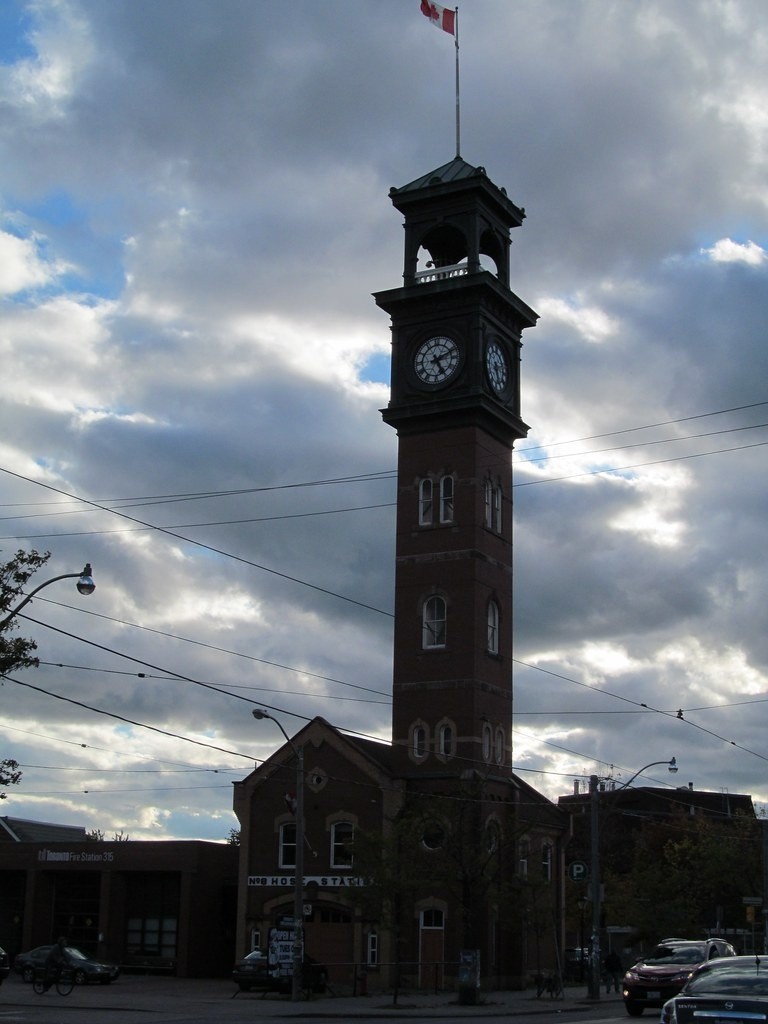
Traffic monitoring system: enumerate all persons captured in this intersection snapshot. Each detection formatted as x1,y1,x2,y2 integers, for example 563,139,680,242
604,947,623,994
44,935,76,991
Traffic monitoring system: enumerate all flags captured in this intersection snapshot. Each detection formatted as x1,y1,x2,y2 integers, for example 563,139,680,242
420,0,455,37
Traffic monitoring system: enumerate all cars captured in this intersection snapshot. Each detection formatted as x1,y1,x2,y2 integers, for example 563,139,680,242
15,946,118,985
233,948,328,992
661,955,768,1024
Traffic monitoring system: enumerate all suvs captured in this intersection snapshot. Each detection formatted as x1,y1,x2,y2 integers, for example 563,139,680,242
622,938,736,1016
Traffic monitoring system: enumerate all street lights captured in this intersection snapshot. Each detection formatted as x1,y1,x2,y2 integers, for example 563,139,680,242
587,756,678,998
253,708,304,1002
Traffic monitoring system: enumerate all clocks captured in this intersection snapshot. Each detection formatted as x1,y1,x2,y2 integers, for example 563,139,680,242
482,332,517,404
403,324,468,393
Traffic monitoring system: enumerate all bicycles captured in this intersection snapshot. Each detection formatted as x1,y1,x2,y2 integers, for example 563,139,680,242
33,960,76,996
535,972,562,999
